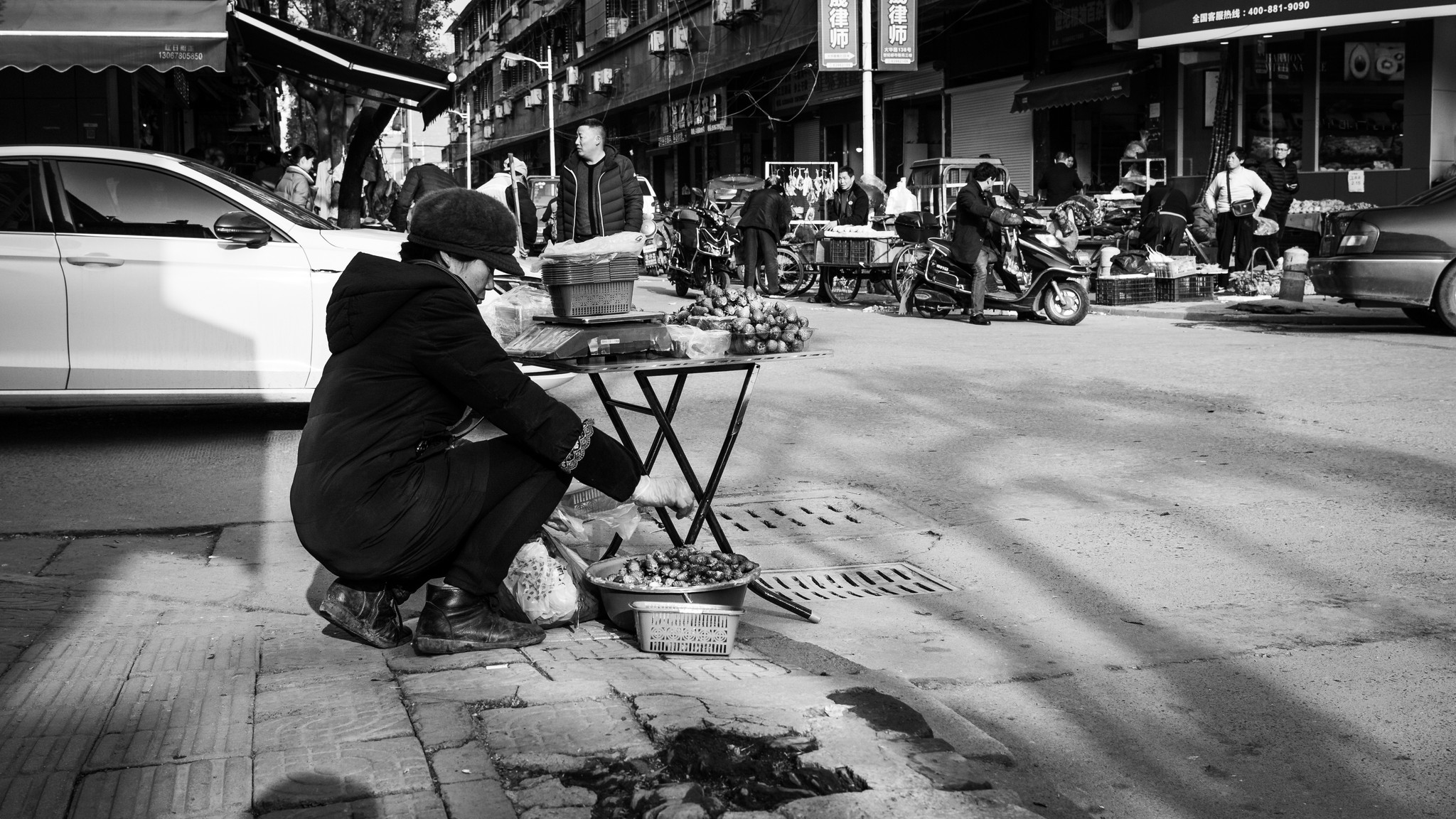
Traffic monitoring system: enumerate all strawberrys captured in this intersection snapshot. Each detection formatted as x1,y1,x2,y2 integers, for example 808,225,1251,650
631,304,644,312
604,544,759,587
656,282,811,354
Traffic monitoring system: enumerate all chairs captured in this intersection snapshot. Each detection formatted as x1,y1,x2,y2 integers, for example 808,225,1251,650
1183,227,1218,264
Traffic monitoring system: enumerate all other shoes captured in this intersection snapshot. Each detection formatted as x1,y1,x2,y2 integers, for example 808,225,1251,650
808,297,831,303
769,290,786,298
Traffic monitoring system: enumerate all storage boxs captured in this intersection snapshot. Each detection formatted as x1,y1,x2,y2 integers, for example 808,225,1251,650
540,255,641,319
894,211,942,244
831,236,908,262
1095,255,1216,305
726,215,741,237
671,210,701,231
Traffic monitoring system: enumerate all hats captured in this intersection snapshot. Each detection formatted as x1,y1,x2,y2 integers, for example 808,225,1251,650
503,158,528,188
407,188,525,277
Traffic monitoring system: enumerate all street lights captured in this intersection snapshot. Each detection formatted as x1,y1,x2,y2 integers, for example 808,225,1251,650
448,102,472,190
502,46,557,198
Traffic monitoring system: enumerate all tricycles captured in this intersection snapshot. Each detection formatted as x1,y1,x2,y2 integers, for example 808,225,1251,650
729,234,816,298
812,199,957,304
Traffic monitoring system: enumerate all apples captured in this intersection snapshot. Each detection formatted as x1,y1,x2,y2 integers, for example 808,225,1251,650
1288,199,1379,214
1320,136,1394,171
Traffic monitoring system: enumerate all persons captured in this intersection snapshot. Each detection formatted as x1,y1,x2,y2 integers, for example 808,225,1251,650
283,117,1303,325
290,186,696,653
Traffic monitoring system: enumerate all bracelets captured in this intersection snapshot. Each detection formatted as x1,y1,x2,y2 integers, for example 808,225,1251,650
558,417,597,473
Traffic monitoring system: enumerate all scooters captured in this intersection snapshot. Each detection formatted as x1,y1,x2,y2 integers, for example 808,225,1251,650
906,184,1095,325
640,186,741,297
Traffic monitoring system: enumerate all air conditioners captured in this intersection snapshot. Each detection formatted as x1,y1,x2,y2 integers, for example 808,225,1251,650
672,25,689,51
712,0,734,24
617,18,630,35
449,3,582,141
734,0,756,14
590,68,614,95
648,31,665,54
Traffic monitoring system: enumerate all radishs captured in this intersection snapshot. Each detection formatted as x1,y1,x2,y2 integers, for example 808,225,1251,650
1141,243,1175,269
833,224,877,232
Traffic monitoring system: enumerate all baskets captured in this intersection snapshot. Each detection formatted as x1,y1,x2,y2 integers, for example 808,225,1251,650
1146,255,1197,279
629,602,745,655
1235,248,1279,296
1156,274,1214,302
564,484,626,520
541,251,639,317
1096,278,1156,307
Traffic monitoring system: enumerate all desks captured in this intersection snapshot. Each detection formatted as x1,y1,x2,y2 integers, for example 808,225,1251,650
1278,212,1324,252
507,348,835,624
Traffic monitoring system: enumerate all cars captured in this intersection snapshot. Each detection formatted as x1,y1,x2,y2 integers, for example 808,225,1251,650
0,144,422,406
1308,176,1456,332
633,176,658,221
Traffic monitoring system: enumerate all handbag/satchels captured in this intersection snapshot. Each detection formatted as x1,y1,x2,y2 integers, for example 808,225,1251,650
1136,211,1160,245
1253,216,1279,235
1230,199,1256,217
503,541,601,630
1109,249,1151,275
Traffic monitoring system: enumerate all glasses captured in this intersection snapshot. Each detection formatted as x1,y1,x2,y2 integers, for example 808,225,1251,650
1275,147,1289,151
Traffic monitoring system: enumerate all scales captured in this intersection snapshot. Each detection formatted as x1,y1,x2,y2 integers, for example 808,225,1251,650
501,309,682,363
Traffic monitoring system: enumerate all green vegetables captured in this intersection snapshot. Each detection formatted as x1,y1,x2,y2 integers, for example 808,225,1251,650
1228,268,1284,295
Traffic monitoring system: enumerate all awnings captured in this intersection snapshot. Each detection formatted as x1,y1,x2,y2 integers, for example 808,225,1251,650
0,0,450,115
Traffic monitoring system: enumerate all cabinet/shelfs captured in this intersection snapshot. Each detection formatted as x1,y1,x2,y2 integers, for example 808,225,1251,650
1118,157,1168,202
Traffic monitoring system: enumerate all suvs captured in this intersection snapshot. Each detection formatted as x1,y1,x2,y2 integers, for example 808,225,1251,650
525,175,562,248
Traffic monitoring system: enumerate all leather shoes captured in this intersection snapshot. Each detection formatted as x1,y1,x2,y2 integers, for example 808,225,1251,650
969,313,991,325
1017,311,1047,321
319,577,412,649
416,582,546,654
